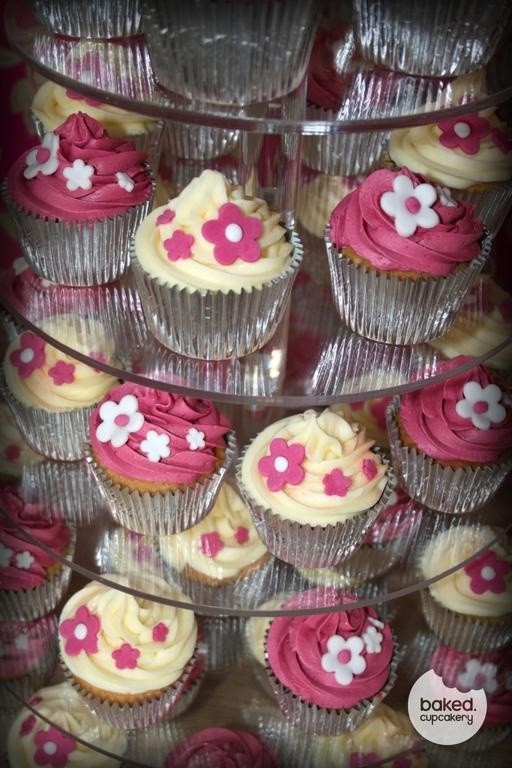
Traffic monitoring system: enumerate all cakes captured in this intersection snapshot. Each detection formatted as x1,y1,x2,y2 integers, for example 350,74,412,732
1,16,511,767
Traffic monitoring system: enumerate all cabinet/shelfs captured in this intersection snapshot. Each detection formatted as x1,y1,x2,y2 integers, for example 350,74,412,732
1,3,512,763
